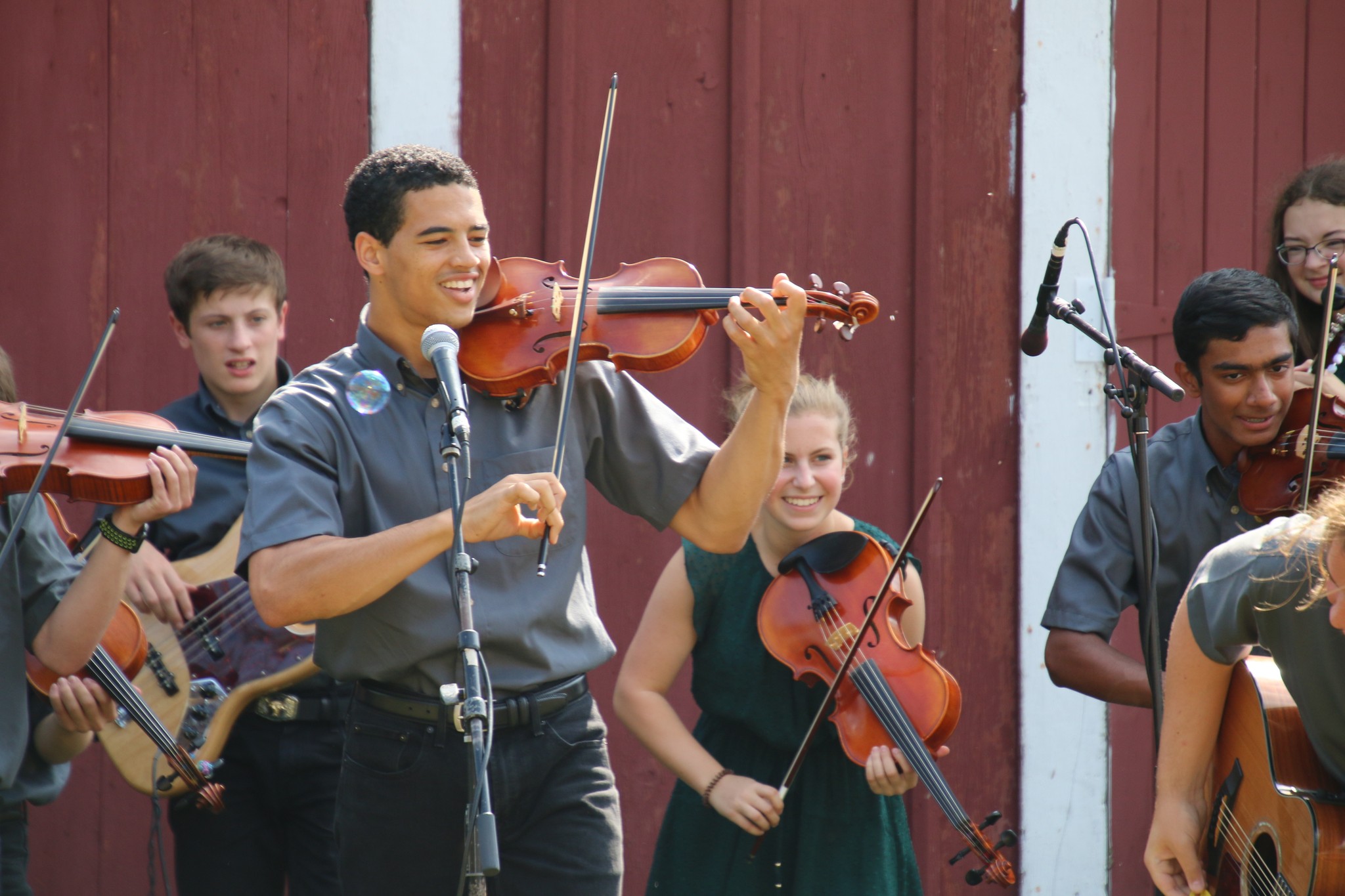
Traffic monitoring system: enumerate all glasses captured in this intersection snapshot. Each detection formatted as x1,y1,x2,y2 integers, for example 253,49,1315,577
1276,237,1345,265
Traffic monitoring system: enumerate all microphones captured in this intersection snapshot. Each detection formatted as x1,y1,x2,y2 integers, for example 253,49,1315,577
1022,226,1069,356
420,323,471,444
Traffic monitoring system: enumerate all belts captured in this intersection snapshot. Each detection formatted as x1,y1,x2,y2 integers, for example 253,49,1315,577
352,672,590,730
245,694,336,725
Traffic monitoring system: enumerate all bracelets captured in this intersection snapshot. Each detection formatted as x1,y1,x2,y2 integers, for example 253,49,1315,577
701,768,735,806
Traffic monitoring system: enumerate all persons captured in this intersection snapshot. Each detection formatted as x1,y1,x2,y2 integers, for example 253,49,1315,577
77,234,350,896
1141,479,1345,896
1,343,198,896
610,370,951,895
1261,161,1345,416
235,147,807,895
1041,267,1303,713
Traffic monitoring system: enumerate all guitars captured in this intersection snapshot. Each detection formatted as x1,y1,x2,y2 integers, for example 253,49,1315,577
1209,655,1344,896
94,513,327,796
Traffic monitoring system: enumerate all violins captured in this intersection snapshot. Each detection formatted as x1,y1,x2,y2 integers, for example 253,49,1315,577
1,401,252,508
21,493,229,817
1237,388,1345,524
457,253,879,396
757,529,1018,891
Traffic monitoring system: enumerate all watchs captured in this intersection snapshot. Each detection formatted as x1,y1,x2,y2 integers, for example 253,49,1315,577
99,514,150,555
